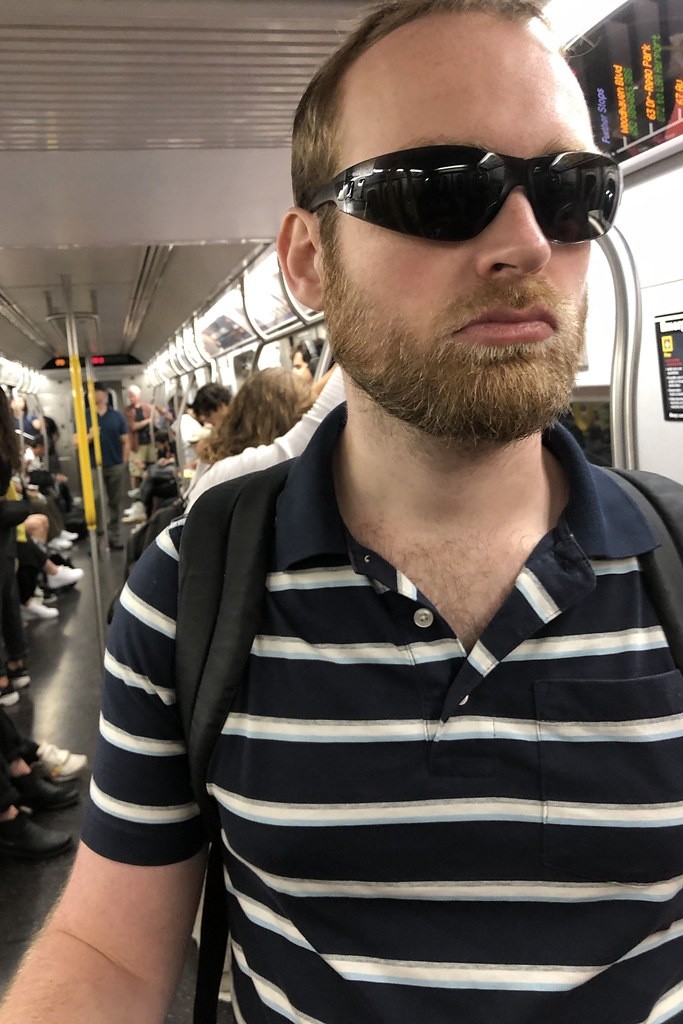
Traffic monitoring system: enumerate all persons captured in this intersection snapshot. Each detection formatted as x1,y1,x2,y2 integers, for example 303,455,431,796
0,0,683,1023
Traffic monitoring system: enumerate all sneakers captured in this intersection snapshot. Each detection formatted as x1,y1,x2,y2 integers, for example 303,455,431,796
34,743,88,781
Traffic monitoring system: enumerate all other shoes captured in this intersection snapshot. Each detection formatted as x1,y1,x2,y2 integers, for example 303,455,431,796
21,527,85,625
2,809,73,857
122,487,147,535
1,665,30,708
16,771,82,807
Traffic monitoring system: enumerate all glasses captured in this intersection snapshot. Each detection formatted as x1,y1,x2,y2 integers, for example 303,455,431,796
306,146,622,242
